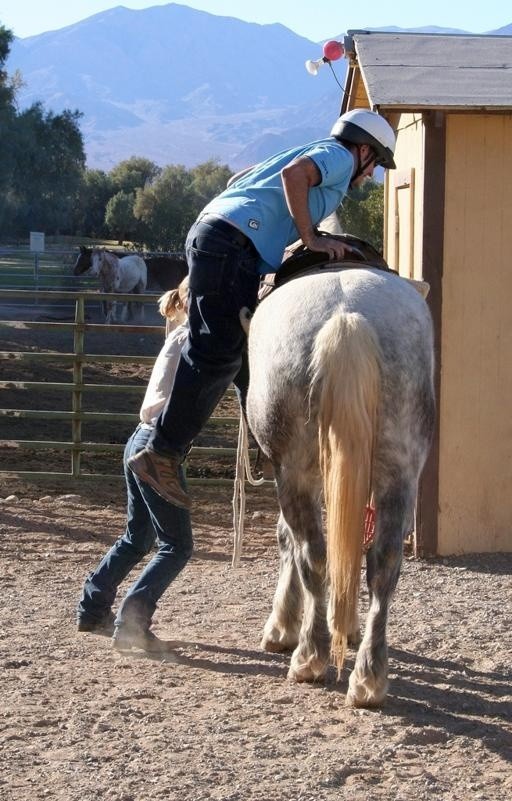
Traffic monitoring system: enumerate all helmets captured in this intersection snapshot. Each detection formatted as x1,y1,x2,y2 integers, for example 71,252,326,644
330,109,397,170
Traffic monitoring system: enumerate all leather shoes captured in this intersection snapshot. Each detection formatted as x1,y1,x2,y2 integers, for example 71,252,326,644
76,614,116,631
111,624,181,653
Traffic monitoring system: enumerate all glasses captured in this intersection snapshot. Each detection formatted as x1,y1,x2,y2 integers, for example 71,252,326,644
370,144,381,169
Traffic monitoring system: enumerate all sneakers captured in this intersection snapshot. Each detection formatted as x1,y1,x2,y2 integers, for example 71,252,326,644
125,448,193,511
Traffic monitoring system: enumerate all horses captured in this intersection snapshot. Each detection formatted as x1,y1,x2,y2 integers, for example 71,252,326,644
90,247,147,324
74,245,189,324
247,212,437,706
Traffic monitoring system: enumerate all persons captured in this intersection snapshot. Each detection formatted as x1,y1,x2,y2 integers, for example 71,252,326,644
77,275,192,652
127,109,396,507
